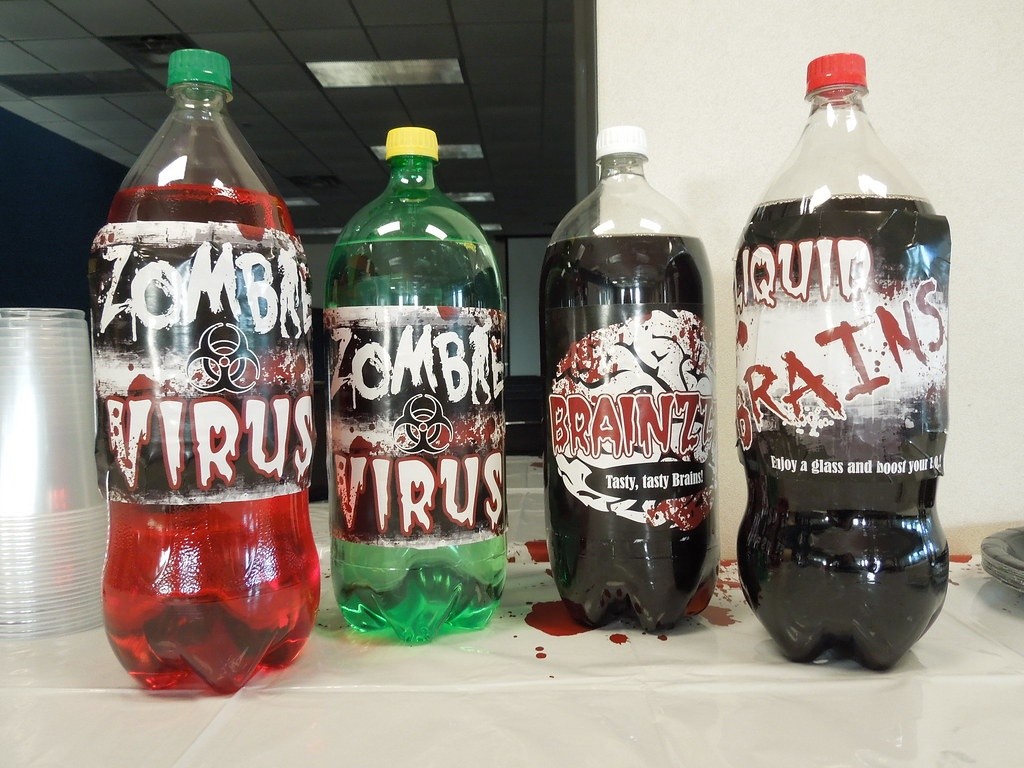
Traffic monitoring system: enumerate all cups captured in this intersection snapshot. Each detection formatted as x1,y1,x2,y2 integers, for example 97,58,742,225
0,308,111,642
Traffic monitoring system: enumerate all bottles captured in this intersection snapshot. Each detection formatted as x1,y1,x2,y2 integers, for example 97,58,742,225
732,54,953,673
540,125,721,633
88,48,323,696
323,126,505,643
